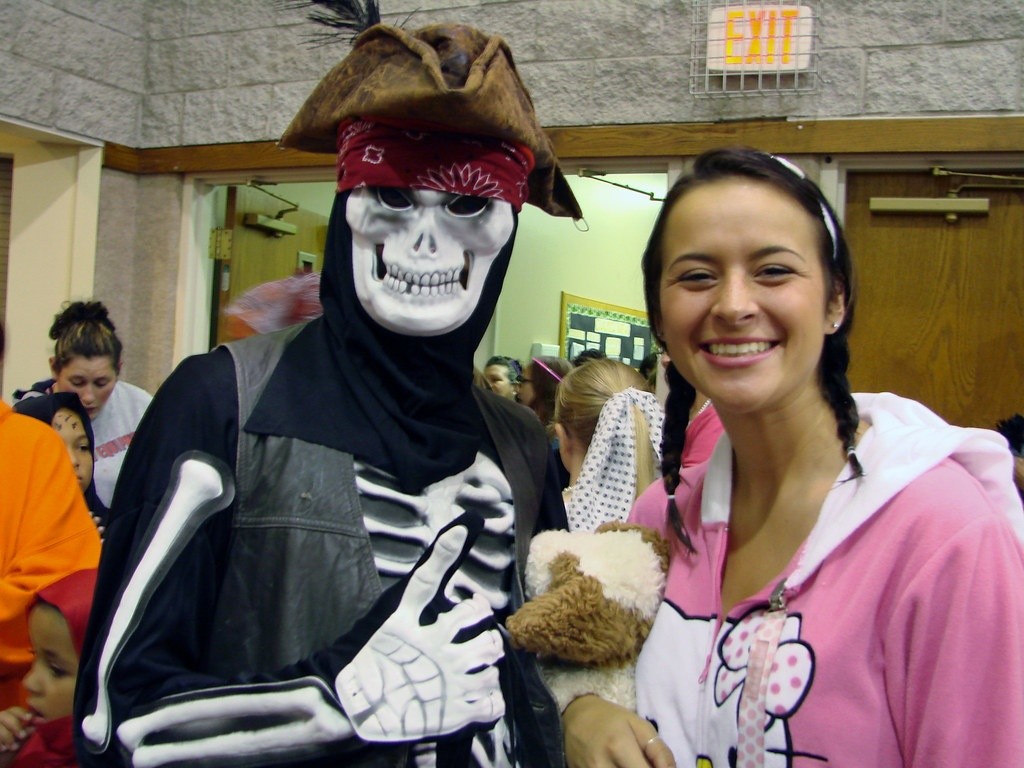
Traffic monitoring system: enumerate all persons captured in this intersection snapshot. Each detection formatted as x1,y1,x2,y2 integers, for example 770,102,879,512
12,390,109,546
49,301,153,509
1,319,102,715
74,28,569,768
474,349,665,534
563,150,1024,768
1,569,98,768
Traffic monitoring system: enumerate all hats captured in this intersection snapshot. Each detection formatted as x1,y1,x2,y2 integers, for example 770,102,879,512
277,1,581,219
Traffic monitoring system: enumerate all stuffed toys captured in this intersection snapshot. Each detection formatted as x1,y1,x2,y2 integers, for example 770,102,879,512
504,520,670,712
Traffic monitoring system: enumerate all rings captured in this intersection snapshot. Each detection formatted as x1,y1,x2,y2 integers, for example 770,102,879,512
644,735,659,752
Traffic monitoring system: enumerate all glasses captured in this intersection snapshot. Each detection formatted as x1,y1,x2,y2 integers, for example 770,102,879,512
514,373,532,388
